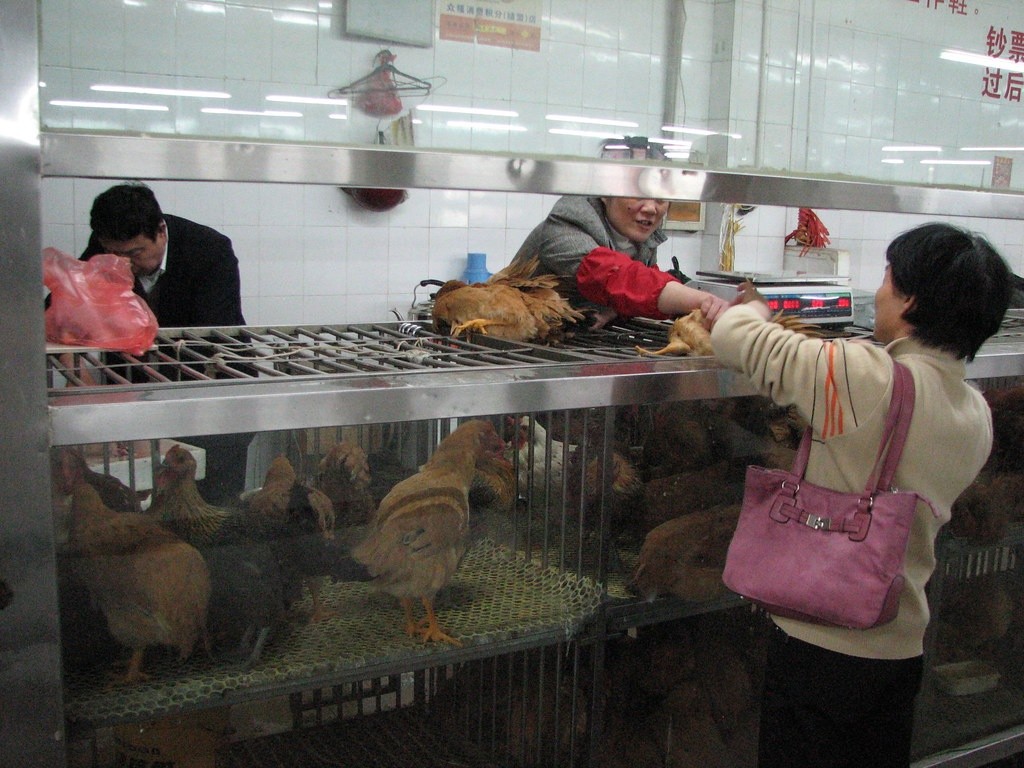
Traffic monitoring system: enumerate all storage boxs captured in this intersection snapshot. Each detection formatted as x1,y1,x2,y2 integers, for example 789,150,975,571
936,657,1000,696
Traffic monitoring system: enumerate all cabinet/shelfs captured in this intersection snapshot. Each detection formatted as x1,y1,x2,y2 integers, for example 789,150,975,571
42,319,1024,768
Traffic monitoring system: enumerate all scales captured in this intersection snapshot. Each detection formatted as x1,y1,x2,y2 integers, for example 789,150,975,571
683,270,856,331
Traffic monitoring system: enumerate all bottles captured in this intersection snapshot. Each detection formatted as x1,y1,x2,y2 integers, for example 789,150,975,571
462,252,492,284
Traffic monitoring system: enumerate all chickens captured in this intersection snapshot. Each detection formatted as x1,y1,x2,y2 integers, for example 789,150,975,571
431,253,598,345
51,395,805,693
634,307,826,357
938,386,1023,664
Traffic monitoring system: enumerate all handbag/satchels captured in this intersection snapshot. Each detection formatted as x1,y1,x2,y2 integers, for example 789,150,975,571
41,246,164,357
722,359,940,631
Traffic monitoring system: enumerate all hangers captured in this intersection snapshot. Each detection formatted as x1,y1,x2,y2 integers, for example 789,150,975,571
340,49,433,93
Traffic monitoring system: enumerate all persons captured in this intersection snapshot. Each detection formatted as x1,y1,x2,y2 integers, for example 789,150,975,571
710,223,1012,768
456,137,730,444
44,184,257,506
